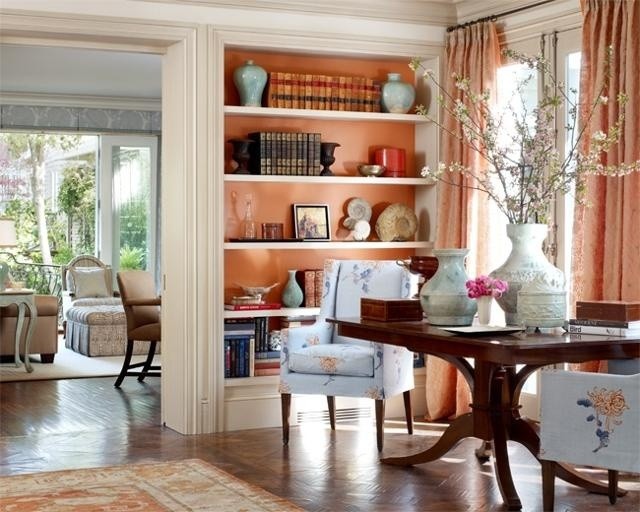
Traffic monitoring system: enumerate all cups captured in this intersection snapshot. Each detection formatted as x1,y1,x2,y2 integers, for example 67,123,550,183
12,282,23,291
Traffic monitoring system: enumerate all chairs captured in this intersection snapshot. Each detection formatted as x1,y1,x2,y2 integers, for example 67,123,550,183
61,255,123,339
114,271,161,387
539,359,640,512
279,259,419,453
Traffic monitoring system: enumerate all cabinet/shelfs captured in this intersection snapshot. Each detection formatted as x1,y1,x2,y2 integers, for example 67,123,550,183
207,30,448,436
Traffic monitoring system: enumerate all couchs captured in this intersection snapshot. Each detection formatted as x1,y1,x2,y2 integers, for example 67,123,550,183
0,295,58,363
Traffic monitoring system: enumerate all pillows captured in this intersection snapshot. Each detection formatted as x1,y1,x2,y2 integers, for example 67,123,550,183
69,268,109,299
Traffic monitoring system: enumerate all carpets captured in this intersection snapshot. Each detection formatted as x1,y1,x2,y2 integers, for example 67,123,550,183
0,458,307,512
0,334,161,382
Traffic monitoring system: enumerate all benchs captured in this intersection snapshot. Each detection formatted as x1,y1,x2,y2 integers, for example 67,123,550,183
65,305,160,357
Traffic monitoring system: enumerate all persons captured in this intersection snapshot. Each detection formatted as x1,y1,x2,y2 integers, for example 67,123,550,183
299,210,318,237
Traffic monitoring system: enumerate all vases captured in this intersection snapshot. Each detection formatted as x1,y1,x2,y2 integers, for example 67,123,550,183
475,295,495,326
420,247,479,326
232,59,269,108
381,72,417,114
486,225,566,326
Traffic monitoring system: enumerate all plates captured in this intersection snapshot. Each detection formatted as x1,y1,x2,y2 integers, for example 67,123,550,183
438,325,525,337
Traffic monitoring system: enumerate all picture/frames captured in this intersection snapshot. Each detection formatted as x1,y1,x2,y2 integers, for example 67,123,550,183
292,203,331,242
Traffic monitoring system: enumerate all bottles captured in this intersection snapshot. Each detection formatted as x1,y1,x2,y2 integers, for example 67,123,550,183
238,199,257,239
269,330,283,352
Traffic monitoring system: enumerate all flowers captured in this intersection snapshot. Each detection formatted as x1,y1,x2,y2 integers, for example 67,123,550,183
408,44,640,224
466,276,508,298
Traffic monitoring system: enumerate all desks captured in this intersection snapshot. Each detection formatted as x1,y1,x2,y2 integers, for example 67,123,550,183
0,287,37,373
326,316,640,510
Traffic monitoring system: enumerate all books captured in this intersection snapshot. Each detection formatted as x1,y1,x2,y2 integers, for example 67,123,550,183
300,269,324,308
567,318,640,339
250,131,321,176
225,301,282,378
267,72,382,111
